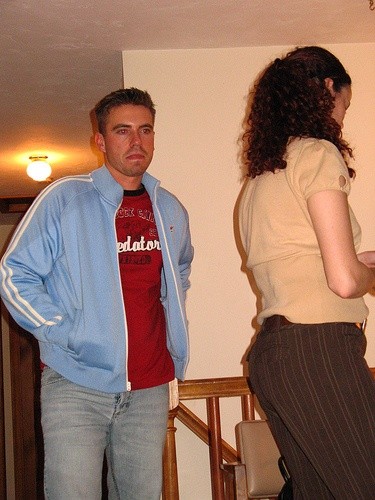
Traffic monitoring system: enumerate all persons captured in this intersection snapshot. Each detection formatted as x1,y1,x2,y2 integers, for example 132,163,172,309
238,46,375,500
0,87,194,500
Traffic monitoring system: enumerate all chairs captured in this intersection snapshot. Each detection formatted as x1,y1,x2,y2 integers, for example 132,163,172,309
219,420,286,500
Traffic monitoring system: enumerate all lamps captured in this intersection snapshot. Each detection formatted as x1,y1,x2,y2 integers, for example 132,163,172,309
26,155,52,182
